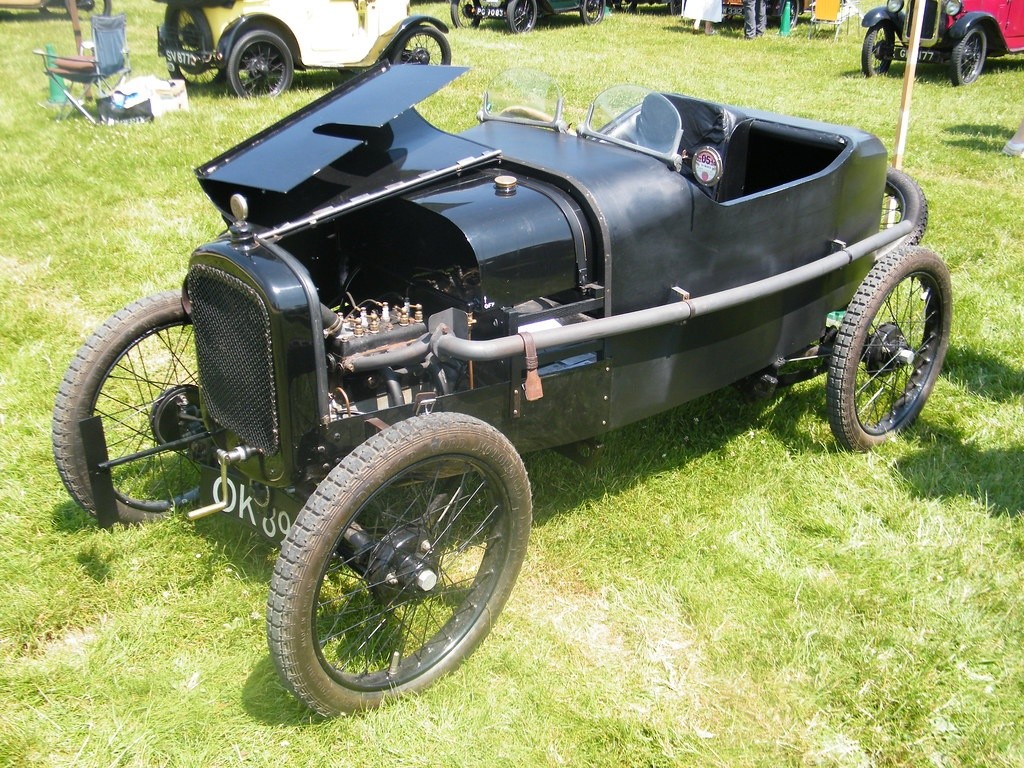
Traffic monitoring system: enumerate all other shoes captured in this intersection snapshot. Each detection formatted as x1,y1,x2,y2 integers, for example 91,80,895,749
693,25,700,35
705,30,719,37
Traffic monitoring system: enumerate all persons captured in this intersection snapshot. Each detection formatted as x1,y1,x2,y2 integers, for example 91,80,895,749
684,0,722,35
743,0,766,40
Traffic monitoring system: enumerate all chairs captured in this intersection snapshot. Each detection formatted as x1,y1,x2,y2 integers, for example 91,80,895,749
718,117,846,203
637,91,734,201
32,11,130,128
809,0,860,44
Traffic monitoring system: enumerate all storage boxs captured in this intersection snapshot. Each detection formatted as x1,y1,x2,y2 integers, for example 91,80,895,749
98,75,188,126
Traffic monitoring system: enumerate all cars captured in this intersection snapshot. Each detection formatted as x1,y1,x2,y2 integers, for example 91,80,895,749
861,0,1024,85
452,0,609,36
49,53,949,721
157,2,453,102
